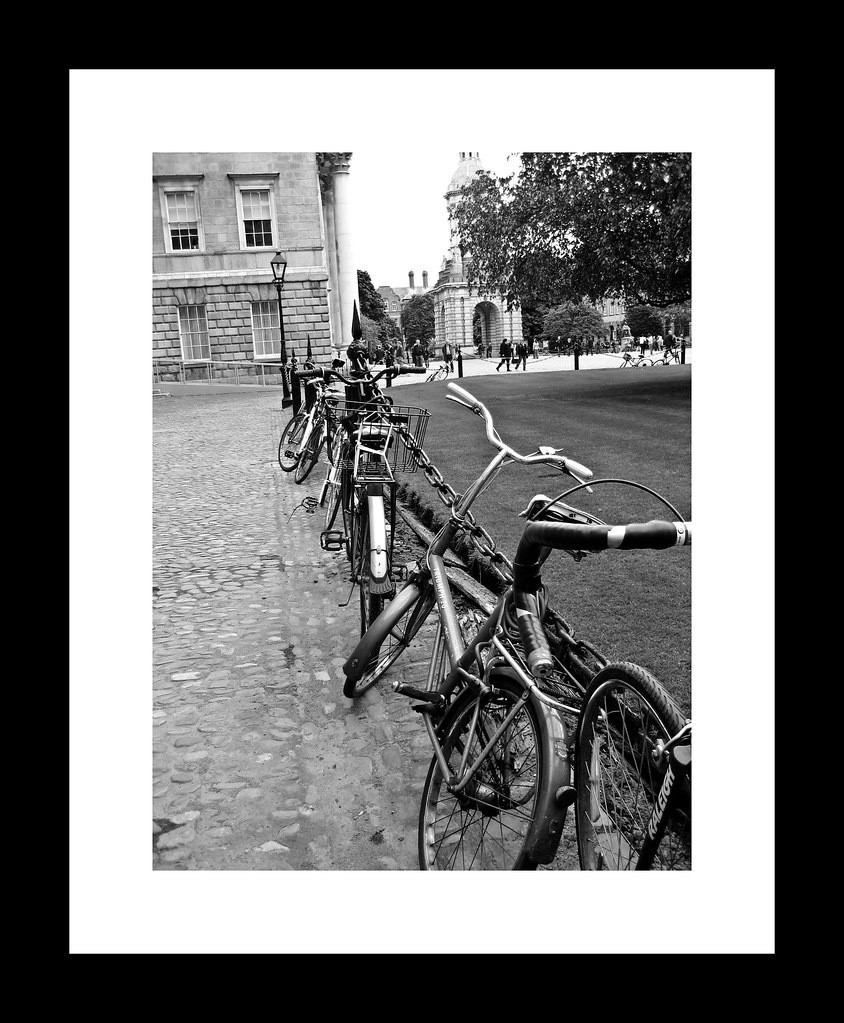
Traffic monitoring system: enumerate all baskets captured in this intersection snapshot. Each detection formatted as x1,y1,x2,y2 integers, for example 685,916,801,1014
323,398,432,474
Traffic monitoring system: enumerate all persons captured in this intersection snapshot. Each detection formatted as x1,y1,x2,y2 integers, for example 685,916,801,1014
533,338,549,359
496,339,528,372
586,330,684,355
371,339,430,367
442,340,454,372
555,336,571,358
487,343,492,358
477,343,485,358
347,329,367,369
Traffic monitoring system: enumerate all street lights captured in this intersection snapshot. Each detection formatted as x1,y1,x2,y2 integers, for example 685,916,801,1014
270,250,293,409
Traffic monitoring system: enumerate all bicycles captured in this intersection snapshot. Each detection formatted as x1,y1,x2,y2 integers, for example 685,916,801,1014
653,344,681,366
512,479,691,870
279,361,432,676
620,351,653,368
426,365,454,382
342,382,592,871
